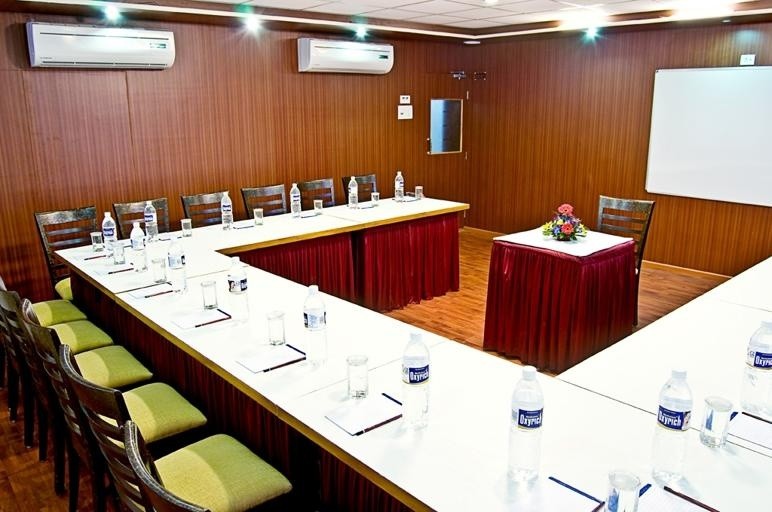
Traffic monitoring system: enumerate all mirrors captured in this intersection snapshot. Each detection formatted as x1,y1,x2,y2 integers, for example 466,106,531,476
429,97,463,156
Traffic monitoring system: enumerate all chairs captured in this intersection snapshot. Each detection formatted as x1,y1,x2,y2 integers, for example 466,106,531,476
1,272,113,512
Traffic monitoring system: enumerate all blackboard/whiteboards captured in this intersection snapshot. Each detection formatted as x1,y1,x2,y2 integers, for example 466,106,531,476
645,65,772,207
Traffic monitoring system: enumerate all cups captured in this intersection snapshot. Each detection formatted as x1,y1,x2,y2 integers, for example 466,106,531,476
203,279,218,310
153,252,165,284
253,207,265,226
91,232,103,251
112,243,126,264
181,219,194,237
267,309,285,346
415,186,423,200
701,397,730,448
348,353,368,395
372,192,380,205
601,469,640,512
313,199,324,214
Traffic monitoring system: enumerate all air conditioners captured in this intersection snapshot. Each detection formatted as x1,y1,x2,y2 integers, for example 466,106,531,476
24,20,176,72
296,36,395,77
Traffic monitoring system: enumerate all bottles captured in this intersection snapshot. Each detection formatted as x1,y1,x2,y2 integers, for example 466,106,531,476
395,171,404,202
348,176,359,208
507,364,543,482
168,233,193,298
290,182,302,218
304,285,327,367
742,319,772,414
220,190,235,231
103,211,119,262
145,199,159,242
227,257,251,323
650,368,694,485
131,223,149,272
403,328,431,428
